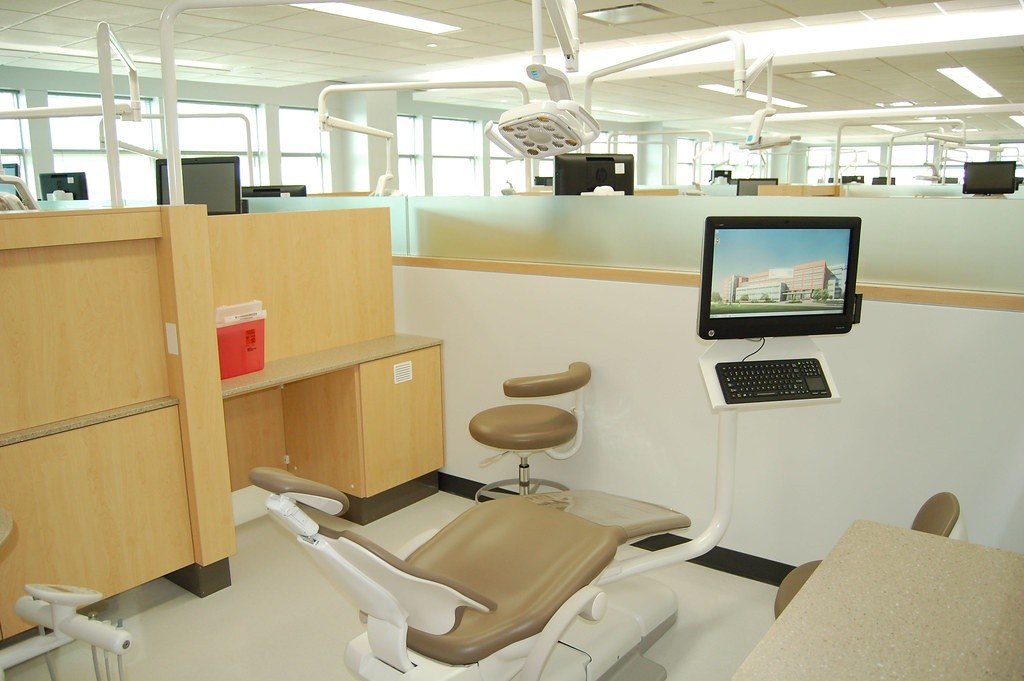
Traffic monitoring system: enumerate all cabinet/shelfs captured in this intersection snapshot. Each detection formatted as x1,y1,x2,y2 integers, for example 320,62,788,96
0,204,443,640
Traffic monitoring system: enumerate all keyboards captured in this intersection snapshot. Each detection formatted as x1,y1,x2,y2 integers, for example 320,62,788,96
716,358,832,405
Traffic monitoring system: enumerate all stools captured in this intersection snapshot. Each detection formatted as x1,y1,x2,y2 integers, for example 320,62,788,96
469,362,591,502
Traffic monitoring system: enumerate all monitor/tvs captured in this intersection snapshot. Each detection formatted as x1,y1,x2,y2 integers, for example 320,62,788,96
698,216,861,340
828,178,840,183
736,178,778,196
842,176,864,184
534,177,552,186
872,177,894,185
962,160,1016,195
155,156,305,215
554,153,634,195
714,170,731,184
0,164,88,206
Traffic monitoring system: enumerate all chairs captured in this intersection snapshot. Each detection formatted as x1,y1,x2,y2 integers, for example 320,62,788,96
776,494,962,619
248,467,691,681
